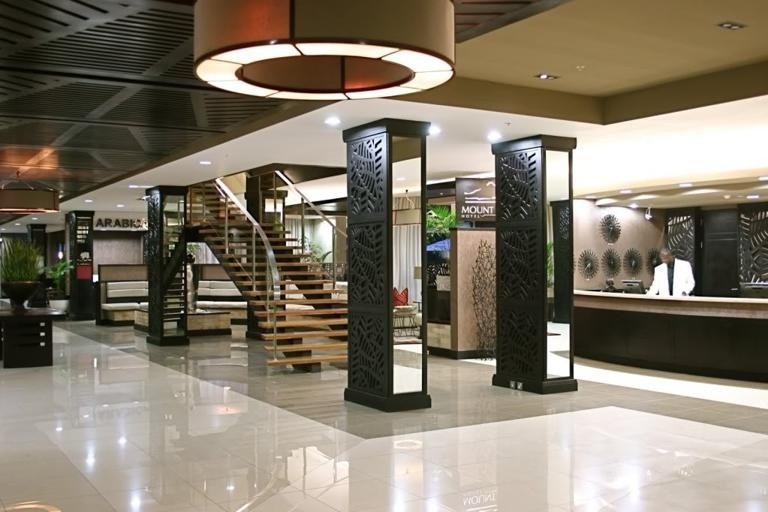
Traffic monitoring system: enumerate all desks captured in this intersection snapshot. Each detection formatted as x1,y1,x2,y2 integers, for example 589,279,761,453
1,300,68,367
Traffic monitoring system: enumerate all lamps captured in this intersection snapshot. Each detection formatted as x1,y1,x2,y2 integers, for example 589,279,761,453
189,1,456,105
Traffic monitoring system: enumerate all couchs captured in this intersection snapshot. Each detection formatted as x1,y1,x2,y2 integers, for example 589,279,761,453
100,281,251,327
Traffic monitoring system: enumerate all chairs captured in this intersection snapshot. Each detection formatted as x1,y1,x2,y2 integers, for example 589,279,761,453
392,287,417,336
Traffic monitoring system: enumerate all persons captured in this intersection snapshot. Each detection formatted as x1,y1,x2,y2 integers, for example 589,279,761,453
645,249,696,297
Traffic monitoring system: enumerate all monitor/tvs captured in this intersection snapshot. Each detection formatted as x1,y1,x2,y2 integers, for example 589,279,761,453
621,279,645,294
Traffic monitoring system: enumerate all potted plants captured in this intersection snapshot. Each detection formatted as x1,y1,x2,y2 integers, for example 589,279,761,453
0,235,45,315
